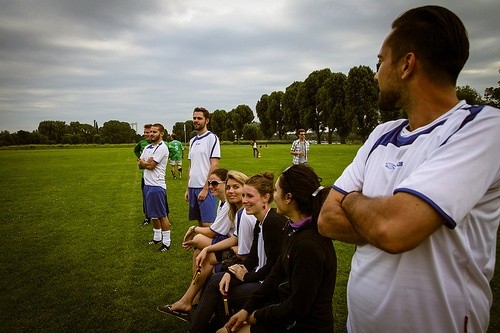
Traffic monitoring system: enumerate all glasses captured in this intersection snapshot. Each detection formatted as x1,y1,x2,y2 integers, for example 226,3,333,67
208,180,225,188
282,163,299,197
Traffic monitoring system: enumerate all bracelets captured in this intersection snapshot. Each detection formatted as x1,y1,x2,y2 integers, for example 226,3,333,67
191,226,198,234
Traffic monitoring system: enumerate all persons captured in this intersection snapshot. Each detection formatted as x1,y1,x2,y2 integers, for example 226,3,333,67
251,139,259,158
182,167,236,310
156,170,258,323
317,5,500,333
169,133,184,179
134,124,180,226
290,128,309,165
214,163,337,333
185,171,289,333
138,123,171,252
184,107,221,228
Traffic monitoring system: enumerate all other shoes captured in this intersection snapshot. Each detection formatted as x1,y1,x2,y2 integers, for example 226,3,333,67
157,304,191,322
148,239,161,245
159,244,171,251
142,220,150,225
